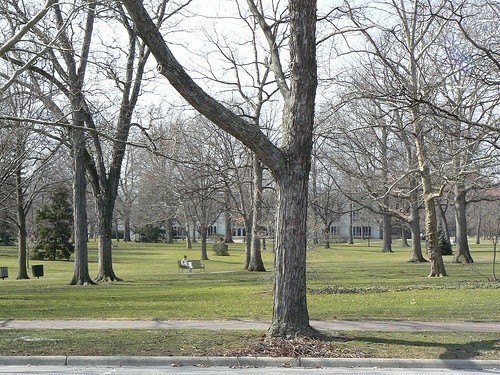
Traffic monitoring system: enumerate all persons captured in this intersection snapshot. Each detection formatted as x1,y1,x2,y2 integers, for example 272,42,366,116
181,255,194,273
419,232,455,245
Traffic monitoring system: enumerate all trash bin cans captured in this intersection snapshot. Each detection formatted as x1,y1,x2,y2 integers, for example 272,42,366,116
32,265,44,278
0,266,8,280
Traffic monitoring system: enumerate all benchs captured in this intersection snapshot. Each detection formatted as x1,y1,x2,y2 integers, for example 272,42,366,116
177,259,206,273
